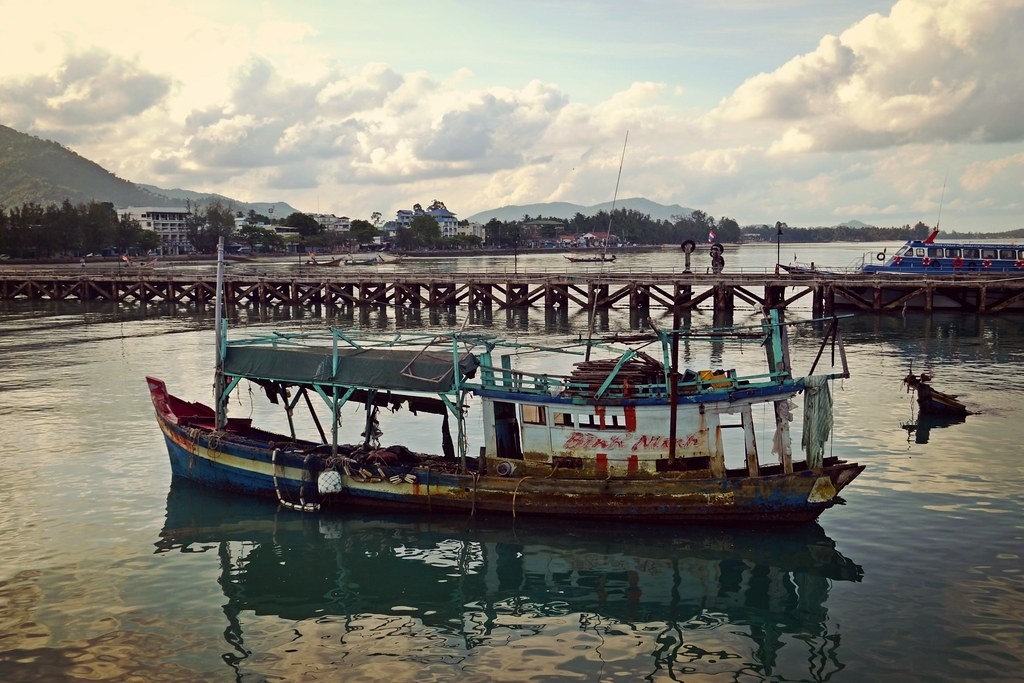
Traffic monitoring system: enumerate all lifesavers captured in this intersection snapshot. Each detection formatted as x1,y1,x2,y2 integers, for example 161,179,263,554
893,256,902,265
681,240,696,253
1014,260,1024,269
931,259,940,267
952,258,963,268
922,257,932,266
969,262,977,270
877,252,885,261
982,259,992,268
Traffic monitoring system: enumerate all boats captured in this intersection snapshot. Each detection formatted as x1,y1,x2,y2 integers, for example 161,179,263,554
143,128,868,521
305,256,345,267
344,257,378,265
561,255,617,262
777,169,1024,311
375,256,402,264
